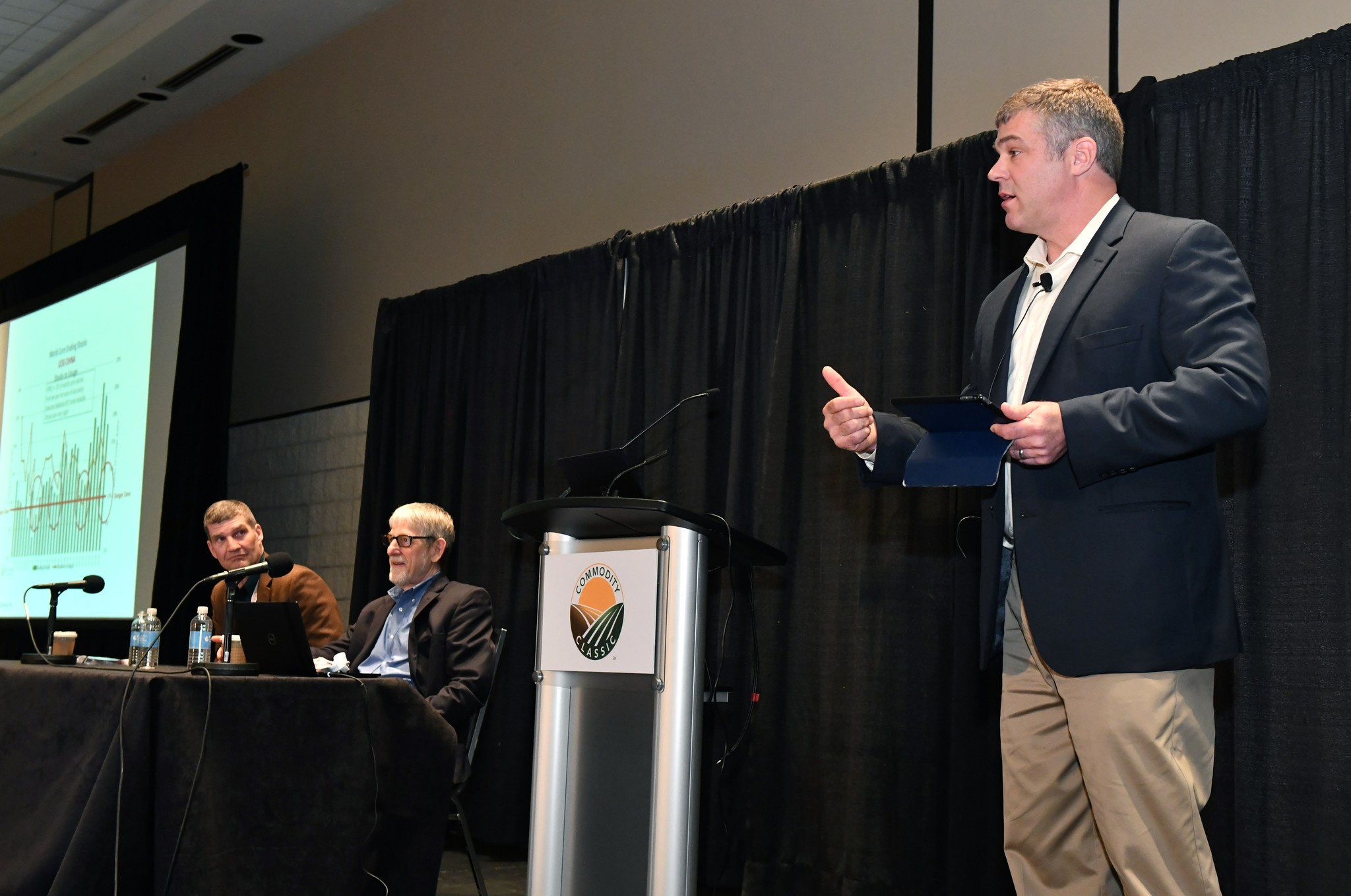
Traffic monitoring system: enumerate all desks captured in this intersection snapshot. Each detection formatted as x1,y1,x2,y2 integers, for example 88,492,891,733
0,659,458,896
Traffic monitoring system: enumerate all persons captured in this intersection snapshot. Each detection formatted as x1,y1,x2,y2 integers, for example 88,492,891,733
211,502,497,896
204,500,343,664
822,75,1272,896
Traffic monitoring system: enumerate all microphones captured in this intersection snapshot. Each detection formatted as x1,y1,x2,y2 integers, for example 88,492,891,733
622,389,720,448
1038,272,1053,293
31,575,105,593
607,451,669,495
205,552,293,582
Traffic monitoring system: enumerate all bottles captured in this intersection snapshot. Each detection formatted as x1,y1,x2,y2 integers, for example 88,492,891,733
136,608,161,670
128,610,147,666
187,606,213,675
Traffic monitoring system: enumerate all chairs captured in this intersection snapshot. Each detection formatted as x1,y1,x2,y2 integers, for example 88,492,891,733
446,628,507,896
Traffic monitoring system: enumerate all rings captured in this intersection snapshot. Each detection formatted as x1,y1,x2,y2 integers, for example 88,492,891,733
1019,449,1025,459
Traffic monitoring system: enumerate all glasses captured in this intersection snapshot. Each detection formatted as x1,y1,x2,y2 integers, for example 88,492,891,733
382,533,436,547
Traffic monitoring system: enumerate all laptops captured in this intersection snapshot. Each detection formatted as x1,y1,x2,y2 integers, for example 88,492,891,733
557,447,643,497
233,601,381,677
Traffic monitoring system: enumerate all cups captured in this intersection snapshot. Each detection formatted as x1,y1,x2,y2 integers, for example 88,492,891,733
52,631,78,655
220,634,246,664
